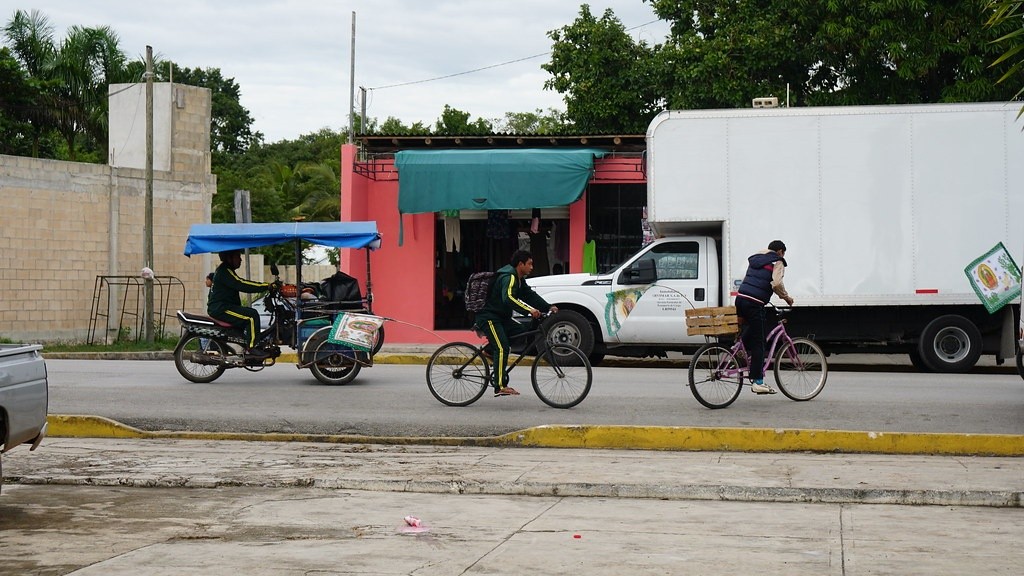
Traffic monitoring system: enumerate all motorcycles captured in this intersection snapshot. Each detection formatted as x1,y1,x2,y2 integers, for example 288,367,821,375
172,221,385,386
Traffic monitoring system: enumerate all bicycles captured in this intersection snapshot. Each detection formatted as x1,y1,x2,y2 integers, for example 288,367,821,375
685,301,828,410
425,308,593,409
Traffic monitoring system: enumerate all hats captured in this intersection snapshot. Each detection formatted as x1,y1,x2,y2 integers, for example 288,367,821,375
206,273,214,282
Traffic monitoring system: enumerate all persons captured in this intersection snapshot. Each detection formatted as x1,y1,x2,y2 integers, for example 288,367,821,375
735,241,794,394
476,251,558,398
201,272,214,349
207,249,281,359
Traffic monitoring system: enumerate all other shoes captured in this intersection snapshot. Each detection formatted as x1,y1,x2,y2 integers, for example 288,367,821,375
245,348,271,358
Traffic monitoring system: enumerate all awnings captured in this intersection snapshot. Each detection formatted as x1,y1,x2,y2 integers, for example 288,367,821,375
395,147,608,247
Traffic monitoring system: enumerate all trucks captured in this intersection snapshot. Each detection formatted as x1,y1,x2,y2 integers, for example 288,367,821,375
510,100,1024,373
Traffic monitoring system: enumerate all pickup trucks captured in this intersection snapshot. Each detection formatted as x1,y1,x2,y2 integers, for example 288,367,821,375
0,342,49,495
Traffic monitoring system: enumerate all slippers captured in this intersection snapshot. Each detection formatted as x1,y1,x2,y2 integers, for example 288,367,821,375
482,350,494,362
494,387,520,397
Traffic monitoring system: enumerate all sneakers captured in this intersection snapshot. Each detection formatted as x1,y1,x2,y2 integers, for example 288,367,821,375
751,383,778,394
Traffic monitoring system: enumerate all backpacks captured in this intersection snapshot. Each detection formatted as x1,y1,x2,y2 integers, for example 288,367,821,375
464,271,519,314
